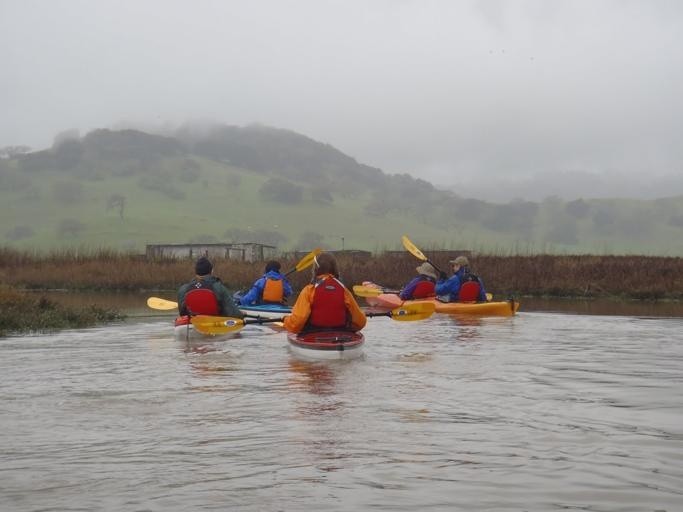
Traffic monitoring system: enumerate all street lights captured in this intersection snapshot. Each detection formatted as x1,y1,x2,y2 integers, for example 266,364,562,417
341,236,344,250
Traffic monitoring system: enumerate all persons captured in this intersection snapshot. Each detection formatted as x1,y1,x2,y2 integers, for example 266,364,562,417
433,254,490,303
397,263,438,301
239,259,293,306
177,256,247,320
281,251,367,333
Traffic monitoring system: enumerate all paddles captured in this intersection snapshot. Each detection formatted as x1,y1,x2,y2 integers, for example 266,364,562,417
147,296,283,327
284,250,321,277
190,302,436,334
402,235,493,300
352,286,404,298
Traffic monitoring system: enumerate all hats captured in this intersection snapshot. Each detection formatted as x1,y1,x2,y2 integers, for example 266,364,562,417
448,255,469,268
195,257,212,276
315,253,339,278
415,262,437,279
266,260,281,273
